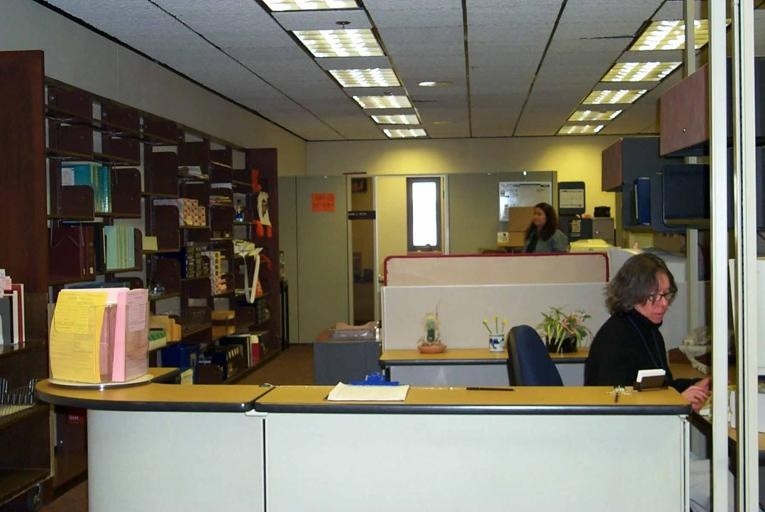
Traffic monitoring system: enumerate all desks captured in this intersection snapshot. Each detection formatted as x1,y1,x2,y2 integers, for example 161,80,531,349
379,348,591,365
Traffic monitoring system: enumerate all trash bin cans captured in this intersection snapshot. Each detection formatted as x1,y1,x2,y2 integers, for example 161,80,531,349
161,343,201,384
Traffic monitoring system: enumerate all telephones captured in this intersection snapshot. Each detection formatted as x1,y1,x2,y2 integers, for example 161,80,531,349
417,311,447,354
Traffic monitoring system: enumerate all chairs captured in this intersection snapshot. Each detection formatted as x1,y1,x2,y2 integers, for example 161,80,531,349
506,325,564,385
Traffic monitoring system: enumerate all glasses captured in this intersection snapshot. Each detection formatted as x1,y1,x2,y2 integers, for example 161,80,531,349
648,291,674,302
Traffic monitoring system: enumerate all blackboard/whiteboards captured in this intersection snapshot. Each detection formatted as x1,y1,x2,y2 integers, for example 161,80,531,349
498,181,552,222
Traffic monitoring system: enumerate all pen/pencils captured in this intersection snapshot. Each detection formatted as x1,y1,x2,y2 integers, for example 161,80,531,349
466,387,514,391
483,316,507,334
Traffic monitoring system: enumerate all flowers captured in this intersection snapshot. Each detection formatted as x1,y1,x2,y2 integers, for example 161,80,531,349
482,314,510,334
533,304,593,353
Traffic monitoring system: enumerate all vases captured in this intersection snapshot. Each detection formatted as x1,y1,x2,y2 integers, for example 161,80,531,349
488,336,504,352
546,335,576,353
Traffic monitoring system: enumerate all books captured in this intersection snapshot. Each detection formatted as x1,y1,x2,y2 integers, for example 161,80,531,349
153,144,233,207
1,267,27,346
148,240,271,384
48,160,136,287
322,381,410,402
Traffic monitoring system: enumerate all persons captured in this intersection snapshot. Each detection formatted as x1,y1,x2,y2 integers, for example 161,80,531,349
583,252,733,412
522,202,570,253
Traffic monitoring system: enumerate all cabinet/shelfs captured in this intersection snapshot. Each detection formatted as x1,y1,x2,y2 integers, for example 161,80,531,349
0,49,279,511
